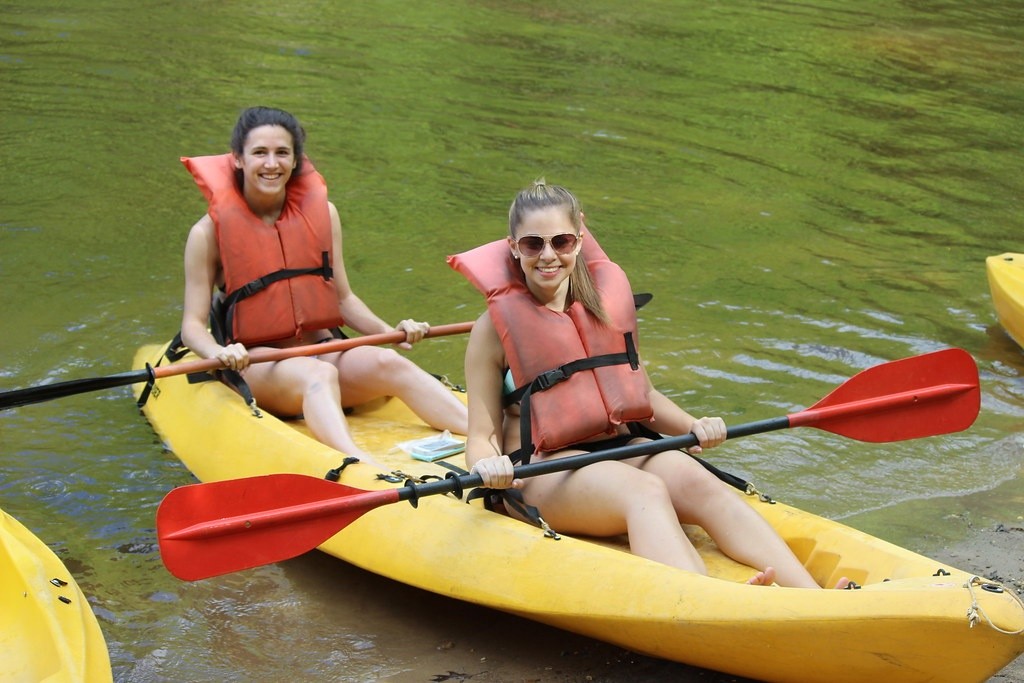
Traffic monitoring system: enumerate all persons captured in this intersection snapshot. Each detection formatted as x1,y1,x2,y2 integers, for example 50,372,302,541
182,105,468,465
466,183,851,589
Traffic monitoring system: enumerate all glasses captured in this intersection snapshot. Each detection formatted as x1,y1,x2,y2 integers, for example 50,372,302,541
510,233,579,257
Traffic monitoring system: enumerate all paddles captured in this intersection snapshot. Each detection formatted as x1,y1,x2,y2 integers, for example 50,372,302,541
156,346,981,584
0,289,656,417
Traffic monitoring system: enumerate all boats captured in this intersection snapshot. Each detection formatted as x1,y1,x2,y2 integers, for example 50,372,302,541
0,506,115,683
130,327,1024,683
983,251,1024,354
